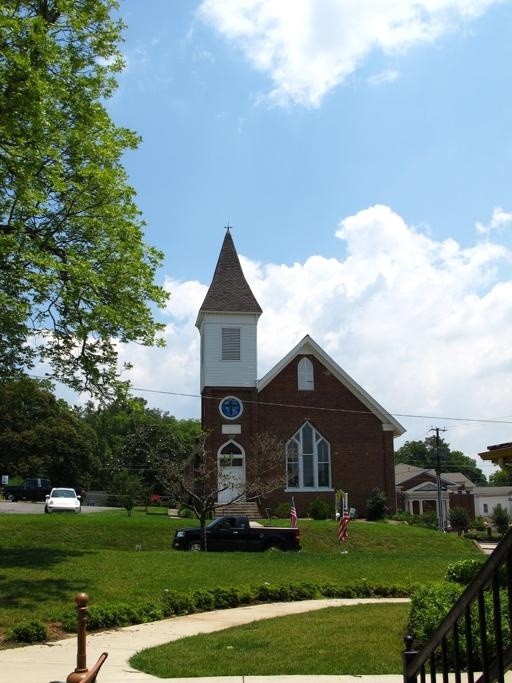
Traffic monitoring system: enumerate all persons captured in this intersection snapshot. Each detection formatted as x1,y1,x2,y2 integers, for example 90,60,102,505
441,518,512,534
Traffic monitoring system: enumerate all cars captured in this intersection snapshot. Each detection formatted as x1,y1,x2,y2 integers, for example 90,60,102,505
45,488,81,512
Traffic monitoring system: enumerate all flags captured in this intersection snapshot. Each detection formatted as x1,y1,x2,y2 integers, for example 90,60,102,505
337,499,347,544
290,497,298,527
343,497,350,538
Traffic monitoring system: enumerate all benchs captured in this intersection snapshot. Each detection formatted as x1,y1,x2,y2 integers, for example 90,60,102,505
213,502,262,521
472,539,495,555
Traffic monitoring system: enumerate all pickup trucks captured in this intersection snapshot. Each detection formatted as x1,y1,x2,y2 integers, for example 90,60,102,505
172,515,302,552
4,478,86,504
